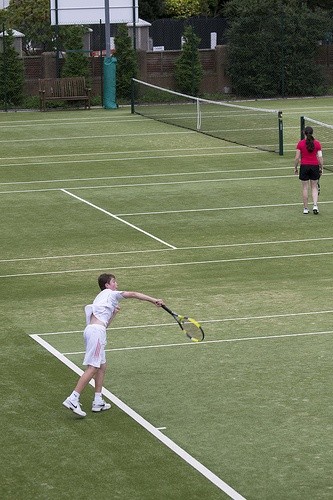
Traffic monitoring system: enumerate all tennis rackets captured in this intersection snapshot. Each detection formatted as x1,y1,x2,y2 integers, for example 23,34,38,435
317,170,322,196
157,302,205,342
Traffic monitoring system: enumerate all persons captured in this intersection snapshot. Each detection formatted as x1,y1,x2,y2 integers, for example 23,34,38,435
62,273,165,416
294,126,323,215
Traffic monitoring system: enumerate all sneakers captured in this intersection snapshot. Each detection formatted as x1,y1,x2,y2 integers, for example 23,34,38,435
313,203,319,214
62,397,86,416
92,401,111,412
303,208,309,214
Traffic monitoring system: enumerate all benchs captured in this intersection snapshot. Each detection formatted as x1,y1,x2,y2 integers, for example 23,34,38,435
38,76,93,110
